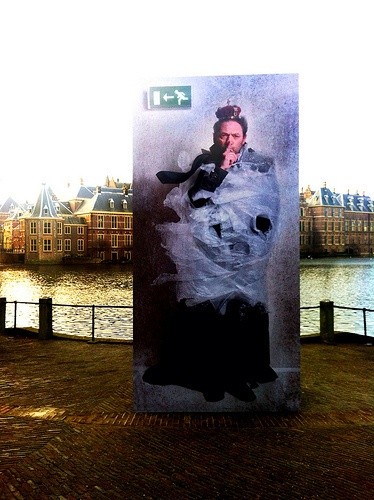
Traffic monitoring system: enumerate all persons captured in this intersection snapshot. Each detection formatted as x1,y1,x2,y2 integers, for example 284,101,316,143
156,100,280,402
